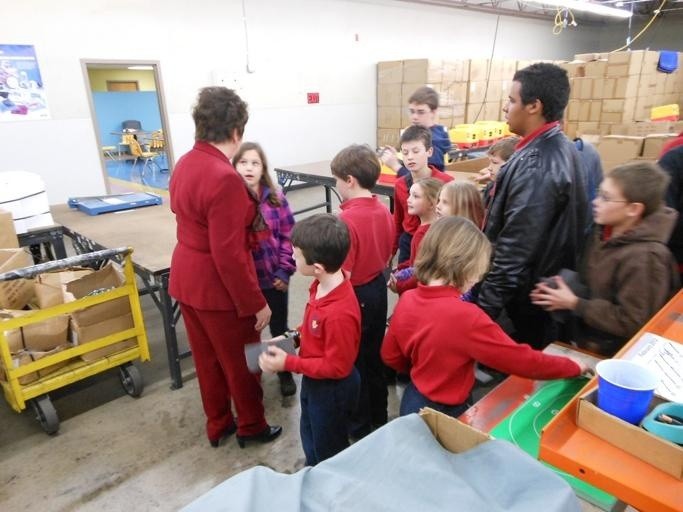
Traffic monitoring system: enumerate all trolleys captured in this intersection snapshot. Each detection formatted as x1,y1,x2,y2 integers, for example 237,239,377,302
0,223,152,437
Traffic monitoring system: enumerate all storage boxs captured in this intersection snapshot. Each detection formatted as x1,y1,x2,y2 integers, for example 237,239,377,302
1,210,138,386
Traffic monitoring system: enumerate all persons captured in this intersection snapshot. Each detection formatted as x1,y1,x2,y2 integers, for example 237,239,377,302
387,176,486,294
476,134,524,205
168,84,283,449
330,145,396,432
475,58,592,401
395,125,454,267
259,214,362,465
529,159,680,362
235,142,298,397
654,130,680,259
380,86,451,261
381,215,595,414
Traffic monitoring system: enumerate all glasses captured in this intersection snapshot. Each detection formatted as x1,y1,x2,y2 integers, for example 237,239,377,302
593,188,628,203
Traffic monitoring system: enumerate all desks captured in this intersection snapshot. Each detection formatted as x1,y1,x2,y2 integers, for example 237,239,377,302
273,160,494,216
49,204,192,391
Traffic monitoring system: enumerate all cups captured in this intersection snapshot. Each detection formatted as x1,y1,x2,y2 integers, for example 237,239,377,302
595,358,660,427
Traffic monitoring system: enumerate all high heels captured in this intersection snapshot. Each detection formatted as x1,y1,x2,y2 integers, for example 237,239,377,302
209,417,237,447
236,426,282,448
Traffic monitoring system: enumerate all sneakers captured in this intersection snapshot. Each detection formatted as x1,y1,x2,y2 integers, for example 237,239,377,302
474,368,498,387
277,370,296,395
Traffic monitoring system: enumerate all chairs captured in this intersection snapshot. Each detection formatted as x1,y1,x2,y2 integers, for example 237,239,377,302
102,129,164,176
